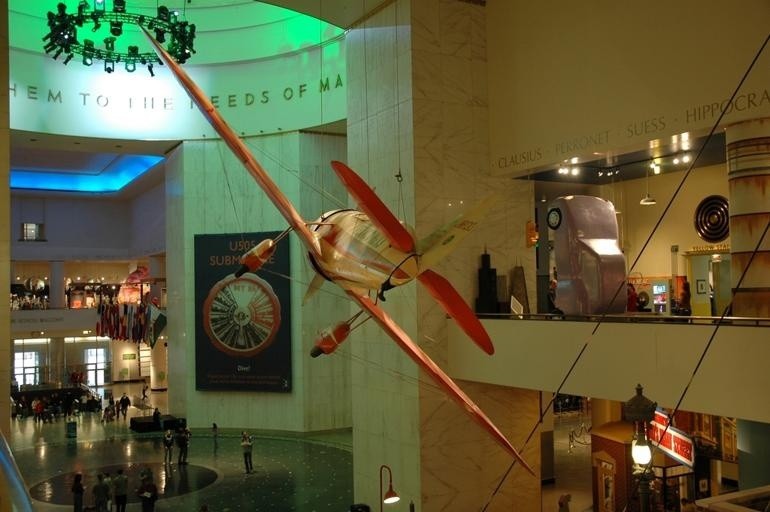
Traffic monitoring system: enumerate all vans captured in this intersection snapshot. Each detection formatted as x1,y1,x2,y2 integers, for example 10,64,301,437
19,381,103,412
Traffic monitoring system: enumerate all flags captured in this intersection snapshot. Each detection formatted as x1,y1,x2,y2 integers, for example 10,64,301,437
97,303,166,349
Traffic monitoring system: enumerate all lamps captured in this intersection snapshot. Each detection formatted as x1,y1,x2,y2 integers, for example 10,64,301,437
37,0,198,79
638,168,658,206
377,462,401,512
557,146,695,179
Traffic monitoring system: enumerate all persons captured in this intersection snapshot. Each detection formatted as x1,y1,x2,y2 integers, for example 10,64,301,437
627,283,637,311
547,280,558,313
11,379,188,512
241,432,253,474
